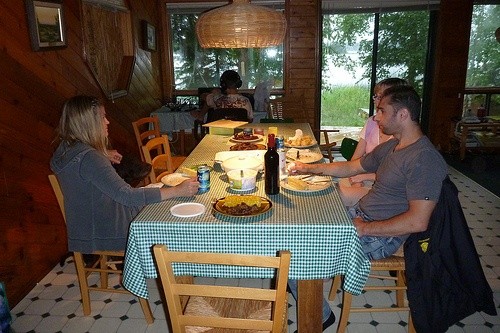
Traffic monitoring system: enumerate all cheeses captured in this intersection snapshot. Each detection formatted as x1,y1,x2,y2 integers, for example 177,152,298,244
287,178,307,190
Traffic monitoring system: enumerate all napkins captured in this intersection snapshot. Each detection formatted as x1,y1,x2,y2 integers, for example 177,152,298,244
289,129,303,140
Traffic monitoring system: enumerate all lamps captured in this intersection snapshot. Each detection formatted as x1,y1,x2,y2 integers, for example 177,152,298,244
195,0,287,48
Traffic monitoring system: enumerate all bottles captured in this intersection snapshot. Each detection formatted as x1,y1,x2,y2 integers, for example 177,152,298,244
276,138,287,179
264,133,280,195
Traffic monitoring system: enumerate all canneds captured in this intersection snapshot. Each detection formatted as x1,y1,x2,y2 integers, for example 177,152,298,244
197,166,210,192
276,136,284,149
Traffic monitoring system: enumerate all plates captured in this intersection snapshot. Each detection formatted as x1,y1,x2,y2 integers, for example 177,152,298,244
280,176,331,193
230,133,263,142
161,173,191,187
462,119,481,124
170,203,205,217
255,142,267,149
286,153,323,164
284,140,318,149
212,195,273,217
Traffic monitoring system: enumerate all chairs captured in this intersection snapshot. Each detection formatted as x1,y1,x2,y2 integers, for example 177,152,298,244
48,87,498,333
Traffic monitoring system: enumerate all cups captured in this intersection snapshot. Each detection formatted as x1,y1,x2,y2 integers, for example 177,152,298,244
269,127,277,136
243,128,252,135
478,109,486,117
254,129,264,135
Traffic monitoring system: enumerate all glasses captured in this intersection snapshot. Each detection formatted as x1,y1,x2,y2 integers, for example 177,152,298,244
372,96,382,101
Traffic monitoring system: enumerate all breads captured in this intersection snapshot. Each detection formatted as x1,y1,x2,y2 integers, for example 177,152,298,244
291,135,312,146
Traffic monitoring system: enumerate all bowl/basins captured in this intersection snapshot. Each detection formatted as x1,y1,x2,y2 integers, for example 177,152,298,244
222,156,263,191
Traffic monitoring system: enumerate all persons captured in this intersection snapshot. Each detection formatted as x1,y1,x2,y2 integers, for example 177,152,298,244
189,69,253,124
50,95,199,251
288,78,446,333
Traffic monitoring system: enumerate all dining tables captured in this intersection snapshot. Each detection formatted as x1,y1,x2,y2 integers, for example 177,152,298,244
120,122,373,333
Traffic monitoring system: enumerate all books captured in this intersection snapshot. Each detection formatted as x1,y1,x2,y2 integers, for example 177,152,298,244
202,119,250,135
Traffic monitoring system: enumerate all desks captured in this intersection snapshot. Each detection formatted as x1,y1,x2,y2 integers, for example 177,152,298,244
148,102,267,156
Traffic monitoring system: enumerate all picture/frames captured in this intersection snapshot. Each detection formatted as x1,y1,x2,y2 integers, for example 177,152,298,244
143,19,157,53
24,0,68,52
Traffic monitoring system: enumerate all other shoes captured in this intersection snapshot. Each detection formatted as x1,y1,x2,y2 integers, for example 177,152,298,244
293,311,335,333
